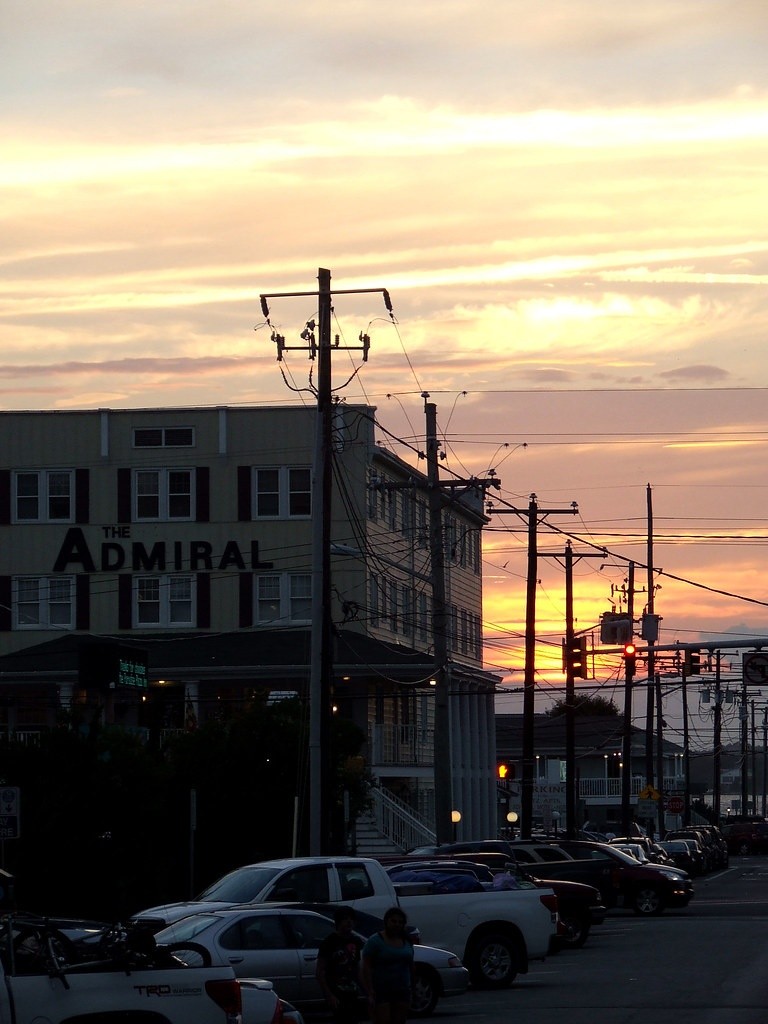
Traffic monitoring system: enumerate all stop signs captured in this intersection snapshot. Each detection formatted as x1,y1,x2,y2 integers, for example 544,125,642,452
667,797,685,813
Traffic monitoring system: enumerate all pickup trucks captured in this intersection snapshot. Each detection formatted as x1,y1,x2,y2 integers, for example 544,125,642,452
0,958,243,1024
129,856,559,989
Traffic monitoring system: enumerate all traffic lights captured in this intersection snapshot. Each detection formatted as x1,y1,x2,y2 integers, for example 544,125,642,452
625,643,636,676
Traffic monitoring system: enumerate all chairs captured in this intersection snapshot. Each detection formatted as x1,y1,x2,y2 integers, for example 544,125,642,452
342,878,372,900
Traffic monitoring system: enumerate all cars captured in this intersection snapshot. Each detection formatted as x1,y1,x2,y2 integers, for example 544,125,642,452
370,819,768,949
153,901,473,1019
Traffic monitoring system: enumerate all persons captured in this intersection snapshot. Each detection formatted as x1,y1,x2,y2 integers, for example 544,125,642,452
313,907,362,1024
361,908,414,1024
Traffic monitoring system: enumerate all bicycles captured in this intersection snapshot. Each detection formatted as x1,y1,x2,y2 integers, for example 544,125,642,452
0,912,212,990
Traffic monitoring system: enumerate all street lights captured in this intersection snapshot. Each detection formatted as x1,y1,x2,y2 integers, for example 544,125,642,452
506,811,519,840
451,809,463,843
